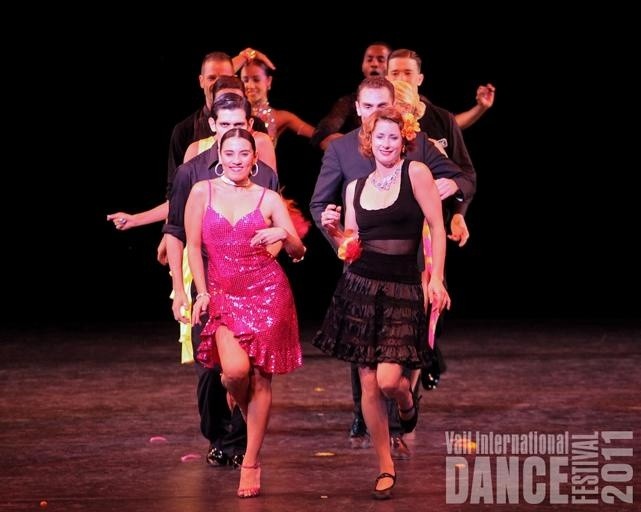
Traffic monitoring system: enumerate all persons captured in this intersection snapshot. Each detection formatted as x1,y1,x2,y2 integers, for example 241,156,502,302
314,105,456,500
106,42,502,466
183,127,309,499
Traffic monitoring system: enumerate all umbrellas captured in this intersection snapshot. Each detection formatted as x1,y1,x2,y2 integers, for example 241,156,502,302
229,453,244,470
390,435,410,460
352,416,366,437
207,447,227,467
372,472,396,500
397,390,417,433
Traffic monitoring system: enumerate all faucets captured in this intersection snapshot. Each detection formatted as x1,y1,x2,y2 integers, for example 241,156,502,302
237,462,261,498
